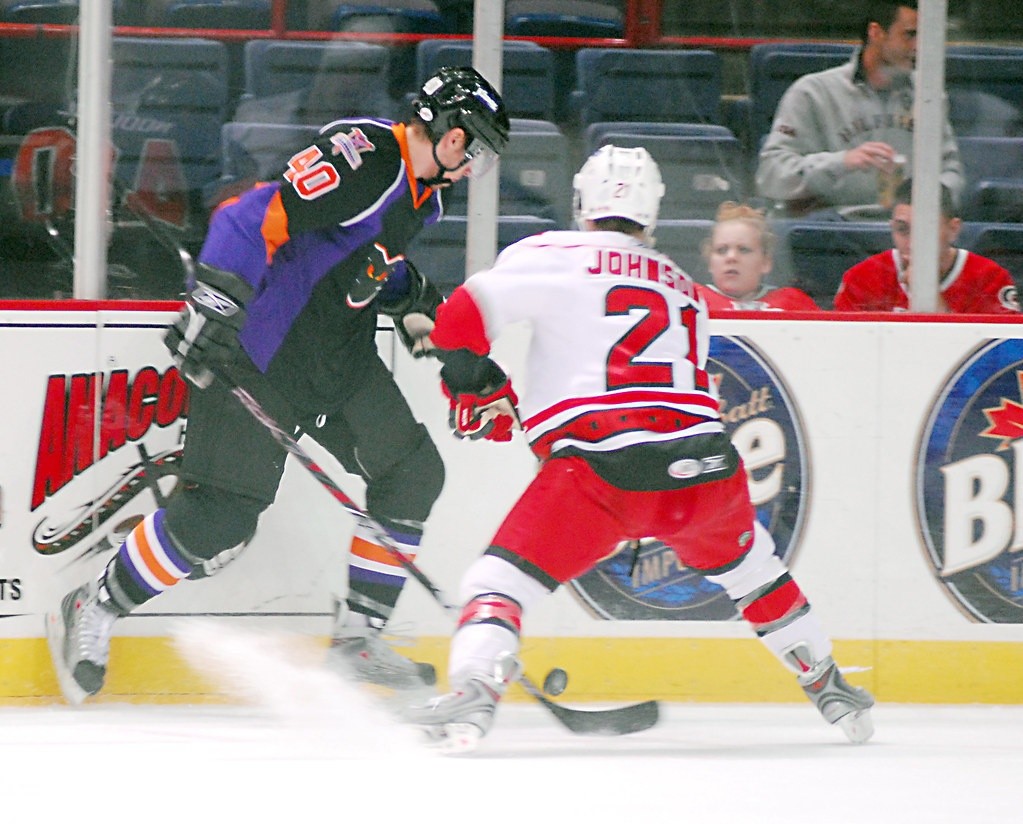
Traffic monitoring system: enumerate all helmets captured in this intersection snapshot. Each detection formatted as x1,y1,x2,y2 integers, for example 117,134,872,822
572,143,665,226
414,64,510,155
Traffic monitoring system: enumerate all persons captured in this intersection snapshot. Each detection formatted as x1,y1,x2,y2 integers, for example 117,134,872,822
402,145,876,747
832,178,1021,314
754,0,965,222
693,201,820,311
43,67,511,703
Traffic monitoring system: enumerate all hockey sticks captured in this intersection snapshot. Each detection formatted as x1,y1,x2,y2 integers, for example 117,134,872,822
215,364,660,737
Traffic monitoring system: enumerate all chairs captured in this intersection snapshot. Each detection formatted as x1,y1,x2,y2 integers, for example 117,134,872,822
0,0,1023,311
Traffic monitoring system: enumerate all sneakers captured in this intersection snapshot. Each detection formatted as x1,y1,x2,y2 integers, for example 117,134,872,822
43,570,120,702
800,666,873,747
400,657,519,752
325,597,436,710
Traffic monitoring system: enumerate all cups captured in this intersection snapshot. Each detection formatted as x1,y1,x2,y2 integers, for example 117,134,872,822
877,154,907,208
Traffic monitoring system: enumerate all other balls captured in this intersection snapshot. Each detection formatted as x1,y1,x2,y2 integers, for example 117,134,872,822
543,668,569,696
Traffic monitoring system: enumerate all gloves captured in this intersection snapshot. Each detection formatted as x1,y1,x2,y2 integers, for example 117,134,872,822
393,271,449,358
164,260,255,391
444,383,519,442
